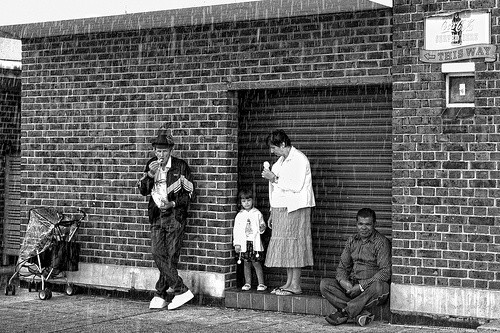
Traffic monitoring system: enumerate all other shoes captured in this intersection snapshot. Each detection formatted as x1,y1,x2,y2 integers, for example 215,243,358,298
168,290,194,310
257,284,267,291
149,296,167,309
242,283,251,290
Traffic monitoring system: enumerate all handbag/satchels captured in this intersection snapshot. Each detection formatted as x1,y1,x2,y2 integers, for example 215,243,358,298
51,240,82,271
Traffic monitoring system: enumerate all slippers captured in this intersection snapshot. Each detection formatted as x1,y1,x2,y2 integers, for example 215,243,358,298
271,286,302,295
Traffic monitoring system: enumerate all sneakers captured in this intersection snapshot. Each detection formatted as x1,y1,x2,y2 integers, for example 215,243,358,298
355,310,375,326
325,307,350,325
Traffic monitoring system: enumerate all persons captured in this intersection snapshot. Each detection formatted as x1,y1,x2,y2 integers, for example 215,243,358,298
321,209,391,326
137,134,197,310
233,188,267,291
261,129,316,296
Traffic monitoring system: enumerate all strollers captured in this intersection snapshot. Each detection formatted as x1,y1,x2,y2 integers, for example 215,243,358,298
3,207,86,300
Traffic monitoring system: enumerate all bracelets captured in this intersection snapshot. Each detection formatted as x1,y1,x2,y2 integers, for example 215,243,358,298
148,171,156,178
172,201,176,207
359,284,364,292
275,175,279,183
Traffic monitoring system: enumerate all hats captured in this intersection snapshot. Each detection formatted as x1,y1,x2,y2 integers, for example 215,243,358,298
151,134,175,146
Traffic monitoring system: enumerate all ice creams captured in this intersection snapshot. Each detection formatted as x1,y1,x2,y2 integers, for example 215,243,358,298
263,161,270,170
